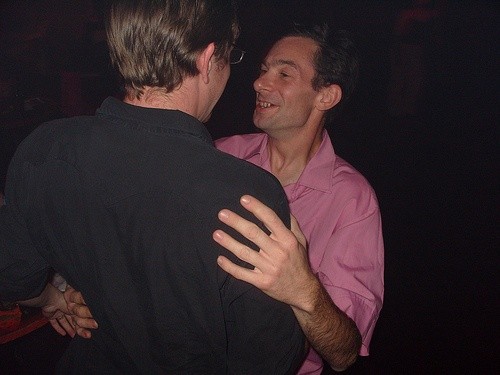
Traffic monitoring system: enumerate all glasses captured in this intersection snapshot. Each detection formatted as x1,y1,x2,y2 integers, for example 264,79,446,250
230,42,246,64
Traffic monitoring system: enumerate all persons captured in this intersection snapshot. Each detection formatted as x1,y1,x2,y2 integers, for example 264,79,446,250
0,0,311,374
66,22,384,375
